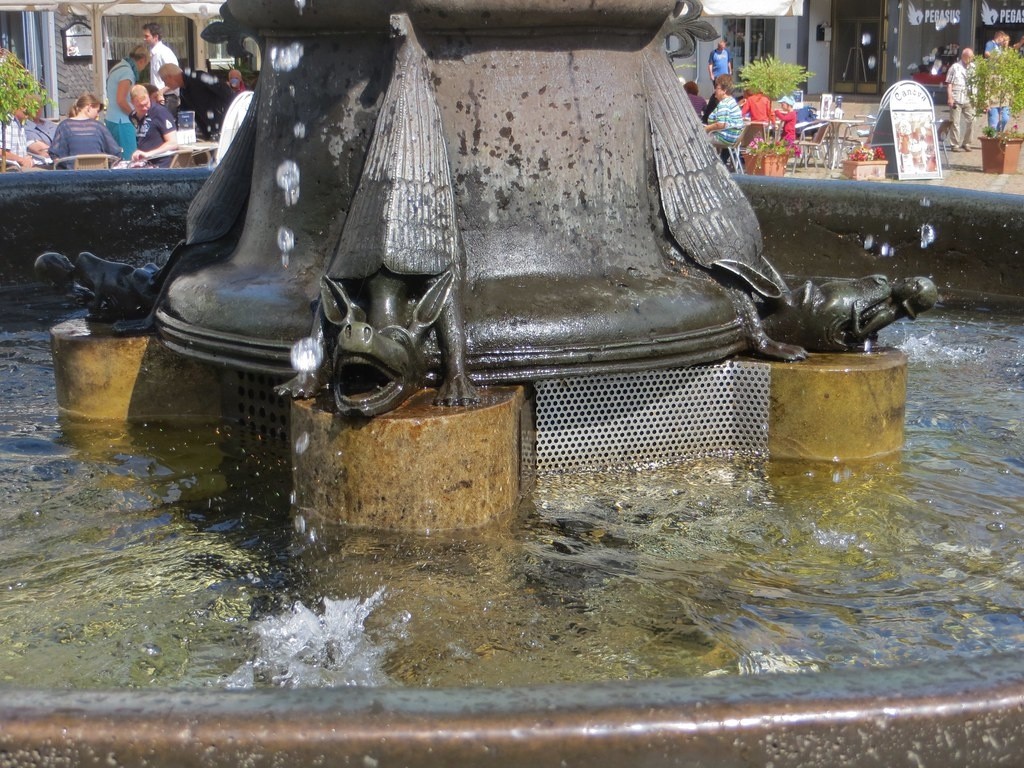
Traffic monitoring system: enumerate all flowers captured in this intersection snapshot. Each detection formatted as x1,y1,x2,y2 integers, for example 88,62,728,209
846,144,887,161
743,134,802,169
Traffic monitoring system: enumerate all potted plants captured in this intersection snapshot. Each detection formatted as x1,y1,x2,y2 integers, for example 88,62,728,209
965,46,1024,174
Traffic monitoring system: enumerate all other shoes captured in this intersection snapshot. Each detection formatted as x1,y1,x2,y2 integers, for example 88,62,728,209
951,146,959,151
962,144,971,152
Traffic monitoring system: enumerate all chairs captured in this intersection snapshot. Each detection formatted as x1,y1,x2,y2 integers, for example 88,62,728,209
52,139,220,171
708,115,878,174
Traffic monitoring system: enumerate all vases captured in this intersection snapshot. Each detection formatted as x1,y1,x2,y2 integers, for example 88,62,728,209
745,156,787,177
843,159,888,180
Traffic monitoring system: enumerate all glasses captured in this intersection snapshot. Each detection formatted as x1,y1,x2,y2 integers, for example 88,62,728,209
715,87,721,91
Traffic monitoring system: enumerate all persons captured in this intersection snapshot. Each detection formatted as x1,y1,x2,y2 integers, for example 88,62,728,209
0,24,247,173
945,31,1024,152
684,41,818,170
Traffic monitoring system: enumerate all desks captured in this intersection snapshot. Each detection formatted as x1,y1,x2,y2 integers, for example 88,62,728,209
821,119,865,170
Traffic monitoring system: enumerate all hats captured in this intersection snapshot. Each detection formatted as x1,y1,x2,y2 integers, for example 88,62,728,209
777,94,795,106
